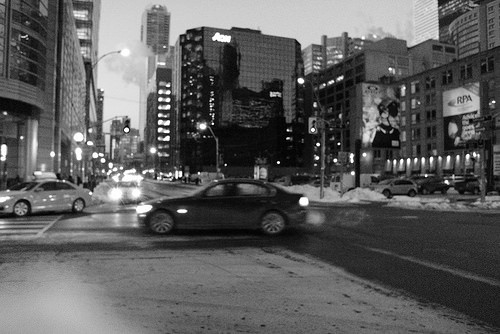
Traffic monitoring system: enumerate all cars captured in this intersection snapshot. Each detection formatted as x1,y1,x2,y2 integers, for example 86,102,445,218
454,174,481,195
374,177,419,198
412,173,448,194
0,179,92,218
116,181,140,203
136,178,310,237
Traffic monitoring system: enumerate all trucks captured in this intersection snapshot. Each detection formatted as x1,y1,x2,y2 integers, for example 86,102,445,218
153,161,173,180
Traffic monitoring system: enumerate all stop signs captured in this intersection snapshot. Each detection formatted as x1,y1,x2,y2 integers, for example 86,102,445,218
337,152,348,162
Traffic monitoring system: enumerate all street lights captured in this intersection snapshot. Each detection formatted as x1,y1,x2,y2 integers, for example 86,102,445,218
85,48,131,136
296,76,327,200
200,123,219,176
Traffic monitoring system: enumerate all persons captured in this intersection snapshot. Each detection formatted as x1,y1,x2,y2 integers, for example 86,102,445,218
14,175,20,184
56,172,96,192
169,174,201,185
223,185,232,195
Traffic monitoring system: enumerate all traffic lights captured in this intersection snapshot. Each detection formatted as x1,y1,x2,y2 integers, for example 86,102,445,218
308,116,318,134
122,118,131,134
